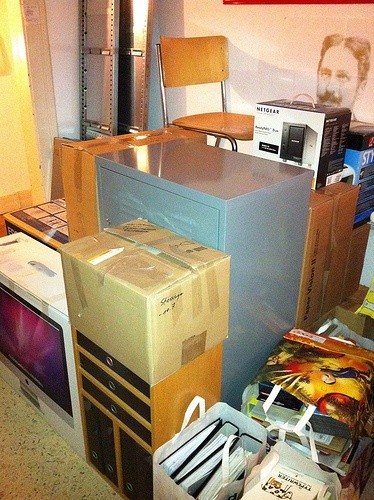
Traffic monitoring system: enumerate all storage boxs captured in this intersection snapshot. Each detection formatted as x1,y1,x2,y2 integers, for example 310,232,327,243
69,323,223,500
296,181,360,331
338,222,371,305
4,196,70,253
0,231,87,464
344,118,373,230
250,93,351,192
58,122,208,242
61,216,229,385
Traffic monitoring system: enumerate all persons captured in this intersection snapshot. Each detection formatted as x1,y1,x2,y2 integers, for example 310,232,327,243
316,32,373,123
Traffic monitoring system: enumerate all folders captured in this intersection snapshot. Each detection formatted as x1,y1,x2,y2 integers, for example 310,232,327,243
159,415,263,500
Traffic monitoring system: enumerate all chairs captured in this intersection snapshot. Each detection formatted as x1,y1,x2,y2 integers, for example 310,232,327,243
155,34,257,151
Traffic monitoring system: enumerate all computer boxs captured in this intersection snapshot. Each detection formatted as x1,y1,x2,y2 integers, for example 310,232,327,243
279,122,318,168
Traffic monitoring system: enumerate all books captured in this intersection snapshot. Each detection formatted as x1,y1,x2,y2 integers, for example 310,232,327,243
240,469,332,500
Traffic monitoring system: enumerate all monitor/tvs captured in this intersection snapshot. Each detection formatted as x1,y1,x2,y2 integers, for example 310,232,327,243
0,282,75,429
